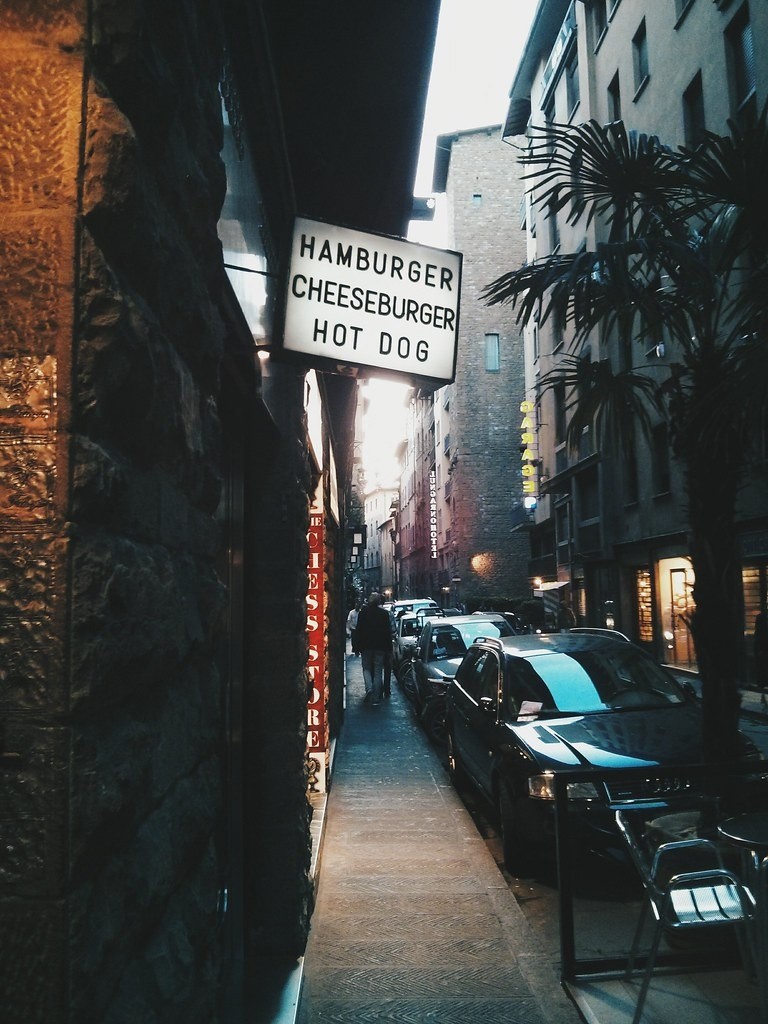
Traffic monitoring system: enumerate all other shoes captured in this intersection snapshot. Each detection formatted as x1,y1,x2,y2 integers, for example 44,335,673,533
372,701,379,705
363,689,373,702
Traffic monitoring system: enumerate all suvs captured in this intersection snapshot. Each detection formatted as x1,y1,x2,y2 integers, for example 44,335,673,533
449,625,767,847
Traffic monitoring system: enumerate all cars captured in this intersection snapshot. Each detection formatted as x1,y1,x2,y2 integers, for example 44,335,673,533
386,600,543,739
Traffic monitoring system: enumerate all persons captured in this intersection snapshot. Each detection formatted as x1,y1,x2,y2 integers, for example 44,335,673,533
557,599,577,632
347,592,398,707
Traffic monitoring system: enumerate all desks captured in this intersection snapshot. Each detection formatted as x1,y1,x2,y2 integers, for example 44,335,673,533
717,812,768,971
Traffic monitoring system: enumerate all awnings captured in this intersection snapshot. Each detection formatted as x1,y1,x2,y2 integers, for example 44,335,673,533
534,581,570,591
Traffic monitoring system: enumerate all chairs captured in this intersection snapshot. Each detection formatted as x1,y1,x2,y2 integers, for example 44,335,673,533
611,808,768,1024
